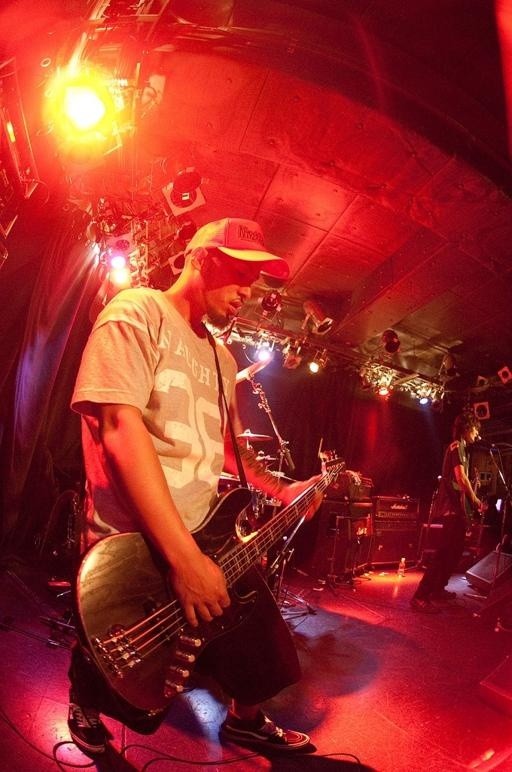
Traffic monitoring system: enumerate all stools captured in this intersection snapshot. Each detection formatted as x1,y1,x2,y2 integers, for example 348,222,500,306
47,579,79,650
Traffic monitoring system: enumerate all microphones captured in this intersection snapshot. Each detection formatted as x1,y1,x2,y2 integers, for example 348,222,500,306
475,436,495,448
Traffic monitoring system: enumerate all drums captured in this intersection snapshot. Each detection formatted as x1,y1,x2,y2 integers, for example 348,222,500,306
216,472,240,494
260,499,283,524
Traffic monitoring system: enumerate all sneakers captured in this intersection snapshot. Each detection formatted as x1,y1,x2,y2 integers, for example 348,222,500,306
410,593,439,614
67,686,109,755
218,708,310,752
431,589,456,600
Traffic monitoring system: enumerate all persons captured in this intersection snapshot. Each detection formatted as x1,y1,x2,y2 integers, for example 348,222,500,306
62,215,332,753
407,411,491,615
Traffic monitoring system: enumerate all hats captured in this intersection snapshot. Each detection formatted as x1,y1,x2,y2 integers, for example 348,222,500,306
184,216,290,262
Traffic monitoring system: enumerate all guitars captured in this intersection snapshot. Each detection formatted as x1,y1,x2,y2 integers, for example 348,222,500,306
463,467,483,559
69,449,347,738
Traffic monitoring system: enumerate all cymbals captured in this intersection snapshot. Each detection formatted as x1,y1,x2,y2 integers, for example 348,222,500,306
255,456,275,464
233,433,272,443
266,471,297,485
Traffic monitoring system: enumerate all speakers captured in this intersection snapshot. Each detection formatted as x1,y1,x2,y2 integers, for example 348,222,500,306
465,551,512,593
371,520,418,566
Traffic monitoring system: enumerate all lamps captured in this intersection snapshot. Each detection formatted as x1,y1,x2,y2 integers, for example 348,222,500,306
227,288,465,405
